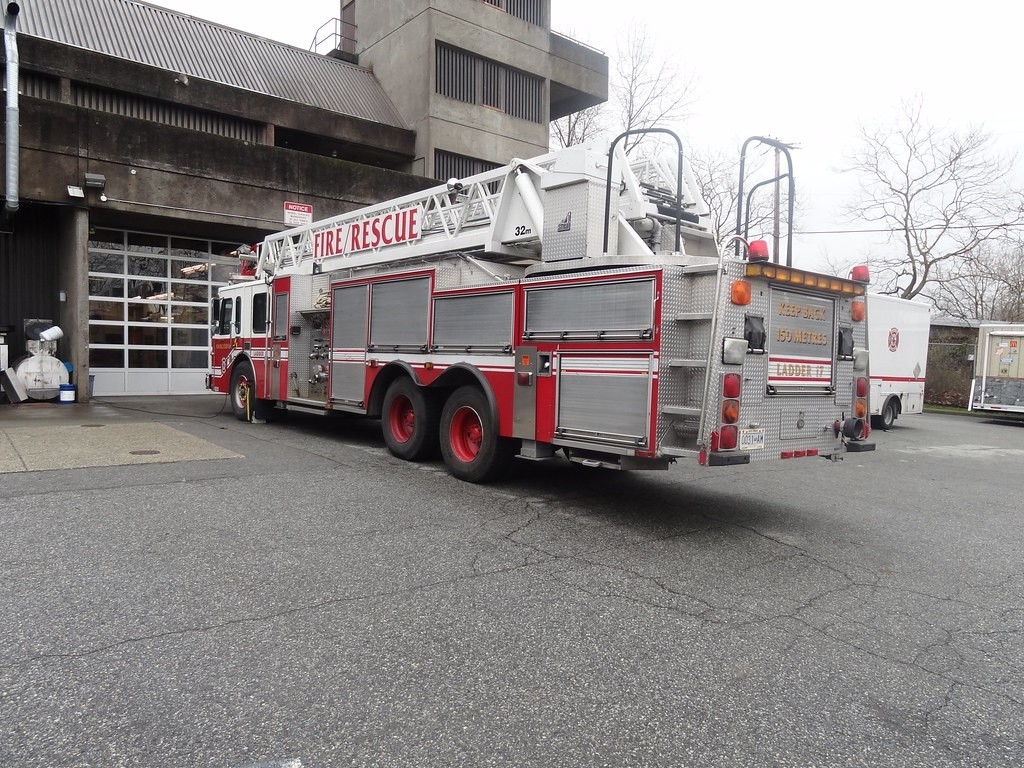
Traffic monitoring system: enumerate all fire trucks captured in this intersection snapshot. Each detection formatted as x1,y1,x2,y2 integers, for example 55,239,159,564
970,324,1023,413
203,126,874,484
868,295,932,431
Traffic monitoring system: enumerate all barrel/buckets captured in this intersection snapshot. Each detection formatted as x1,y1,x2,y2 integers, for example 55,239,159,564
58,384,76,404
